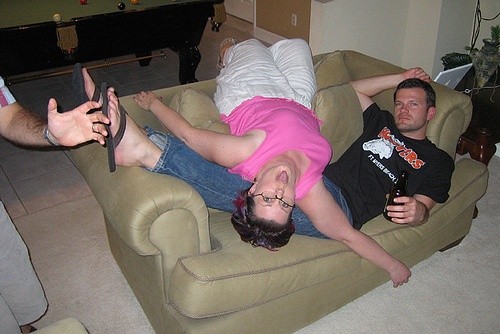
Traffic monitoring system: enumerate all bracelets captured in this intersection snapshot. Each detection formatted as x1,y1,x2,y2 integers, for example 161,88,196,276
44,126,60,147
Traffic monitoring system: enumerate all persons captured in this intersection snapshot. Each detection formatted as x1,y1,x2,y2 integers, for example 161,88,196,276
81,66,455,238
132,38,412,288
0,75,111,334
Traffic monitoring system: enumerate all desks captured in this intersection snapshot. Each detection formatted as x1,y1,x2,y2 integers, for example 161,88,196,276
0,0,215,84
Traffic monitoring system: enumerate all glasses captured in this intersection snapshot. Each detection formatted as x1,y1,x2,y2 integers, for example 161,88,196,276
252,191,296,212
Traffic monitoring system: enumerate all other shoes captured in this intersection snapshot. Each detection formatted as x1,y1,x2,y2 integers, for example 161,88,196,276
215,37,238,72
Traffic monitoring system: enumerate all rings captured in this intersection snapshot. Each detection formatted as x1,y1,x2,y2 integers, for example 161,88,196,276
92,123,95,132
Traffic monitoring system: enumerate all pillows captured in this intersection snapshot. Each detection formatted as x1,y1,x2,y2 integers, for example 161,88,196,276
311,82,363,165
313,50,351,90
168,88,230,135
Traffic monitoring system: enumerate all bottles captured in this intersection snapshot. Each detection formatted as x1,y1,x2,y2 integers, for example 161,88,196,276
383,171,408,221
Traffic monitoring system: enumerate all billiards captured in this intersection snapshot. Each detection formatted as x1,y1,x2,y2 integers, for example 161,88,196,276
79,0,88,6
131,0,140,6
117,2,125,9
52,14,62,22
64,48,75,56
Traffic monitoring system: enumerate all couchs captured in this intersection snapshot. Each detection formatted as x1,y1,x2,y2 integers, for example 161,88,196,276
62,50,489,334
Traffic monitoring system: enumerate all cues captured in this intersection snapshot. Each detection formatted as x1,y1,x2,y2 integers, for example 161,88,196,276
4,50,168,86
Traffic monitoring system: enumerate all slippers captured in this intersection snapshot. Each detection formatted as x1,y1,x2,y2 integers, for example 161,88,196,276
71,62,101,114
99,80,128,172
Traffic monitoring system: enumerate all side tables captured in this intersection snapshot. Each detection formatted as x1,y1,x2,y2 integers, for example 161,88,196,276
449,77,500,216
